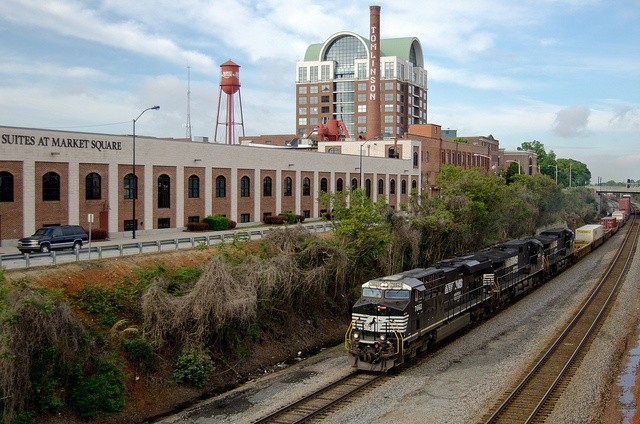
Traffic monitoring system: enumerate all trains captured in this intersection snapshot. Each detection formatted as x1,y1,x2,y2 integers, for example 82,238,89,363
345,194,639,373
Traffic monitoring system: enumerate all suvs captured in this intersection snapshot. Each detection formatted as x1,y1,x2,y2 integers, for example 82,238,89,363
16,223,89,254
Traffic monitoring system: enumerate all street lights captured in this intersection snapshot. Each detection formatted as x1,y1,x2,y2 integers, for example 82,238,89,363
473,153,492,175
548,165,557,184
529,165,540,173
506,159,520,176
133,106,160,238
360,136,379,192
